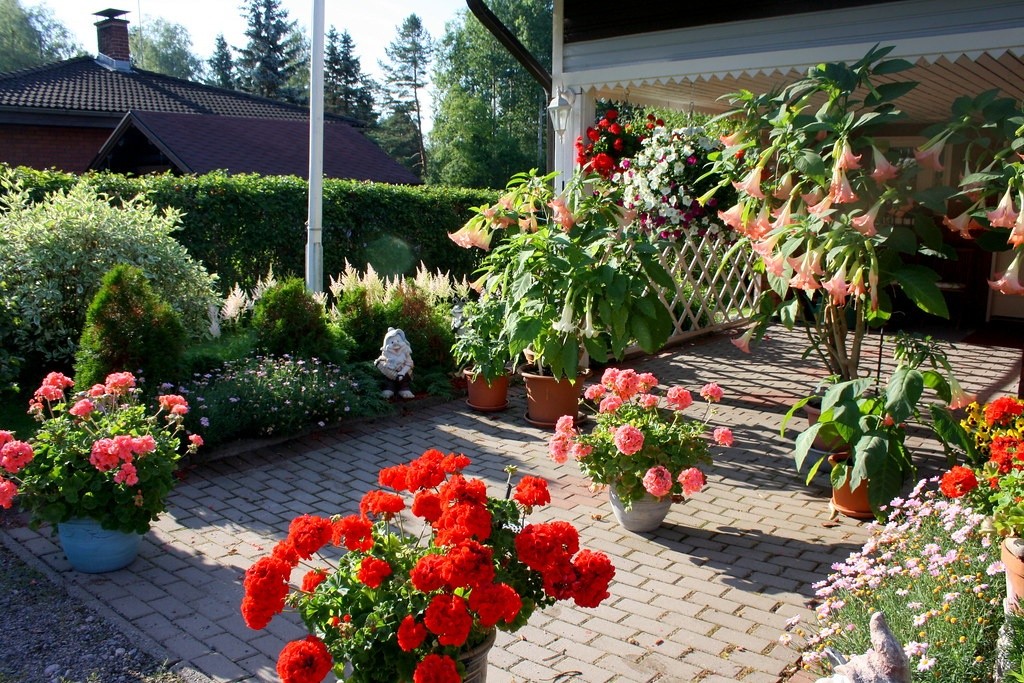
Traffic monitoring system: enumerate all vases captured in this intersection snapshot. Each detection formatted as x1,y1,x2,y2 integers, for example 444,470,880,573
803,397,853,452
58,516,143,573
454,627,496,683
1001,538,1024,602
463,366,513,407
517,362,594,423
828,452,872,513
608,482,672,532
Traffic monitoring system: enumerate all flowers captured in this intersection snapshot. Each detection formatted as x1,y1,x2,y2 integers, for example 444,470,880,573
938,396,1024,537
0,370,204,538
446,42,1024,390
550,367,733,512
241,450,616,683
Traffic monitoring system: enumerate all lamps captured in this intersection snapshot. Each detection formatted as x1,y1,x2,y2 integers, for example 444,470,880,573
547,84,576,144
